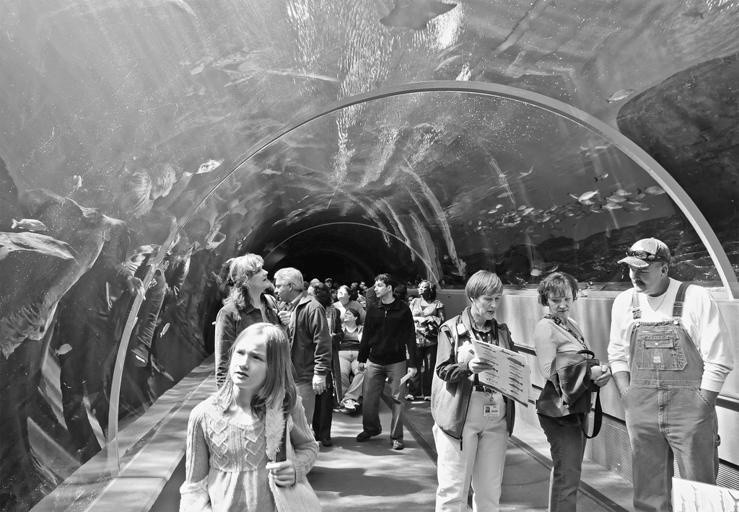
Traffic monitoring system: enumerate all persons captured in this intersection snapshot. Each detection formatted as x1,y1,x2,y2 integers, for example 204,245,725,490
405,279,446,401
306,282,344,447
177,322,319,512
274,266,333,428
393,284,412,306
534,271,612,512
214,254,292,388
332,308,364,415
324,277,333,292
307,278,319,295
355,274,417,450
329,289,338,302
431,270,517,512
350,280,368,308
333,284,366,330
607,237,735,512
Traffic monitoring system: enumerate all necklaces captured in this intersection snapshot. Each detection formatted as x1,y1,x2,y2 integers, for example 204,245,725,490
255,302,264,310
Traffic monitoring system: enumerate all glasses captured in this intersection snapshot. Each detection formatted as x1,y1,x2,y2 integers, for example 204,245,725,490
624,247,669,259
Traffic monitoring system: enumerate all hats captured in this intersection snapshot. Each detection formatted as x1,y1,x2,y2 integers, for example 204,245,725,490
616,237,671,268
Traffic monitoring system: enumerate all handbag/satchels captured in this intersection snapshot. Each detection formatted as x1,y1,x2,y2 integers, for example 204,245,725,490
268,403,324,511
572,349,603,440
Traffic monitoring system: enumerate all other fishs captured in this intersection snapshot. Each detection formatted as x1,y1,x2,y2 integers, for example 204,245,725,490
521,207,535,216
487,209,498,213
517,204,526,210
644,186,664,195
11,217,48,233
127,275,147,301
606,88,636,103
610,189,633,197
604,194,629,203
54,343,72,356
577,189,601,202
497,192,512,199
159,322,171,338
599,202,622,209
593,172,609,182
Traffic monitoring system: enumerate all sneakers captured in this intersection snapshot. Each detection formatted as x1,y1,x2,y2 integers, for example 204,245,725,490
390,437,405,449
423,395,431,402
343,399,356,409
321,436,332,447
351,400,360,409
314,434,321,442
355,428,383,441
404,394,415,400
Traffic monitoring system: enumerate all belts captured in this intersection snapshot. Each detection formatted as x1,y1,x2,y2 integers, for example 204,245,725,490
474,385,495,393
